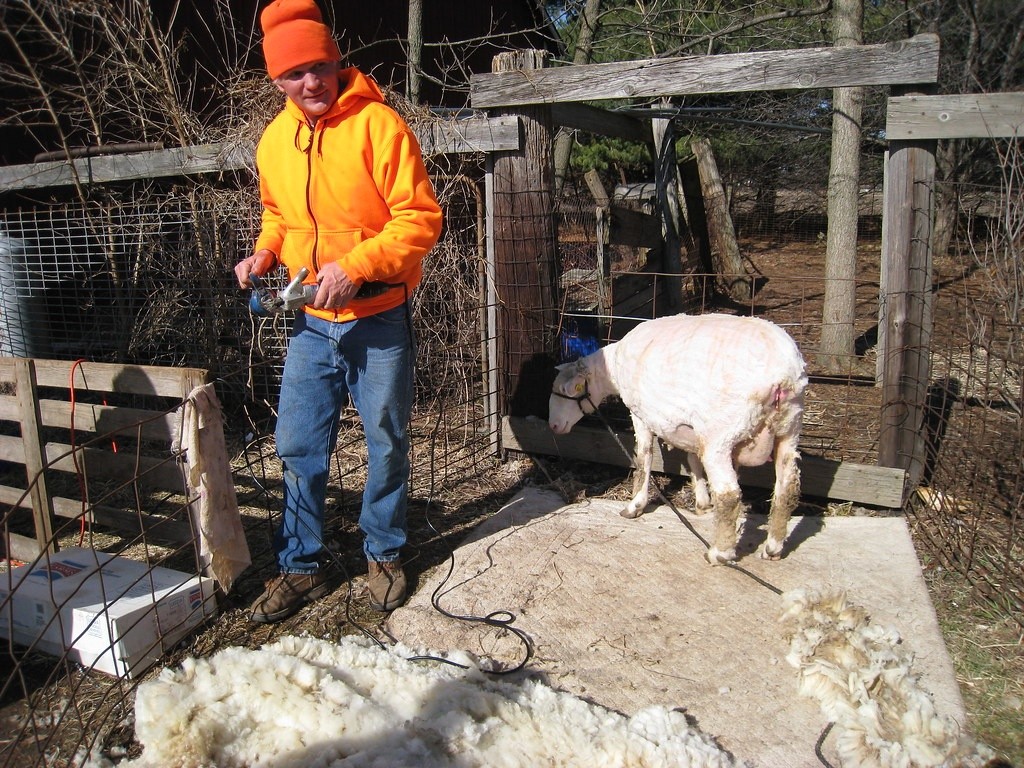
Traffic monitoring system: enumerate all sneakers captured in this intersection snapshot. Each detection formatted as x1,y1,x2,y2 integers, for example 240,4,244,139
367,557,407,610
249,571,329,622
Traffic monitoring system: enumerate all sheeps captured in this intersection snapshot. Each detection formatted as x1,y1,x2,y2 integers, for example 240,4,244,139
548,312,808,567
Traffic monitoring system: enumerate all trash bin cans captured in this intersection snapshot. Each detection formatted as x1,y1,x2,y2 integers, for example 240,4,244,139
558,300,601,363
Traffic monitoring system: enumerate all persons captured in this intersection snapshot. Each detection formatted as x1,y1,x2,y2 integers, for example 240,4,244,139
234,1,443,621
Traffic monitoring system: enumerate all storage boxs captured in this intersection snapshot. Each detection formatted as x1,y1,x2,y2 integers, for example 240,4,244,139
0,546,216,679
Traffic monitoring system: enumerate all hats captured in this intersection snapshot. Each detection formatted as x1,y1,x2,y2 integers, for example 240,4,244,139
261,0,341,78
